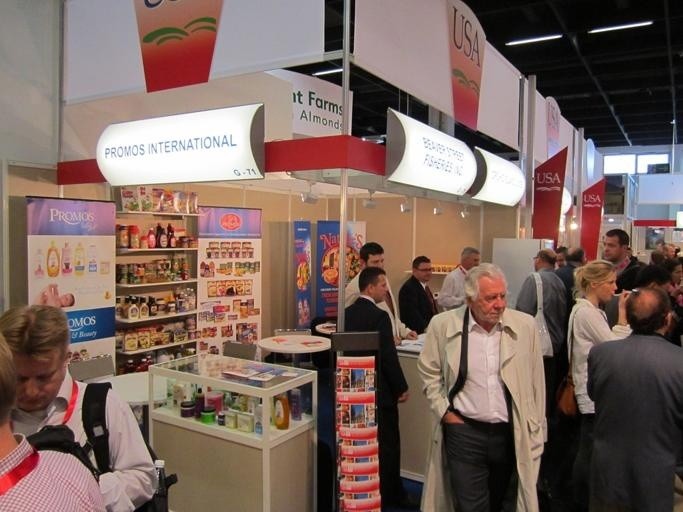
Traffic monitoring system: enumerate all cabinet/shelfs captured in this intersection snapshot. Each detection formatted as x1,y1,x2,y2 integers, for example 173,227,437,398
116,211,199,375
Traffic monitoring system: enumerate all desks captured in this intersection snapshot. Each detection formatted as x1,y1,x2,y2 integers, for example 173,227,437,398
258,334,331,368
395,334,435,484
315,322,338,335
84,371,167,445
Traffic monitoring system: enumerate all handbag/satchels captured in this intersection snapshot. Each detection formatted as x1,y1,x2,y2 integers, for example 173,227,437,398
530,272,553,358
555,374,576,418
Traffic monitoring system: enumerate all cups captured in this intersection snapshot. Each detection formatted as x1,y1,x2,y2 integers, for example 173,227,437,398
322,269,337,283
203,241,255,260
519,227,534,239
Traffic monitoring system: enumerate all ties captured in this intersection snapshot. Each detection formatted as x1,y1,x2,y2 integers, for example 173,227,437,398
425,286,439,314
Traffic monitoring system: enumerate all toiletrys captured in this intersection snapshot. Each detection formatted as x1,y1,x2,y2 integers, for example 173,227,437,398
194,388,205,418
47,241,60,277
74,243,85,276
291,389,302,421
62,242,73,276
205,386,215,406
254,404,263,438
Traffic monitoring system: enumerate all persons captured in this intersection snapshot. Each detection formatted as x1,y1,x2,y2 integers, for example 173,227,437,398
416,263,548,512
0,332,108,512
344,267,420,509
437,247,481,311
515,228,683,512
398,256,439,336
345,242,418,346
35,284,75,308
0,304,159,512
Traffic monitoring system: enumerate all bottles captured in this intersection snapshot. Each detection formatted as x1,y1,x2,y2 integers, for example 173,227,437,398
34,240,96,278
199,261,214,277
175,286,195,311
217,409,224,426
116,222,192,249
125,355,153,373
65,348,88,362
115,294,176,321
193,389,205,420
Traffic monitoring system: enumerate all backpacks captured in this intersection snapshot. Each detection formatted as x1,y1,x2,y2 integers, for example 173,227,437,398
82,382,178,512
